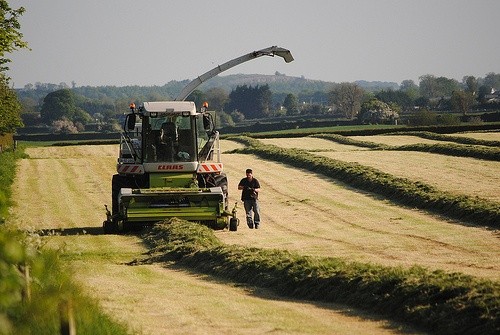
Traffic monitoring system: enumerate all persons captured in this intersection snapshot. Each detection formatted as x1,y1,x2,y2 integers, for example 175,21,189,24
238,169,261,229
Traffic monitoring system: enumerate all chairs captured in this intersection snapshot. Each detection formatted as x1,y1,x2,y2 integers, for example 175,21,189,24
159,122,179,153
